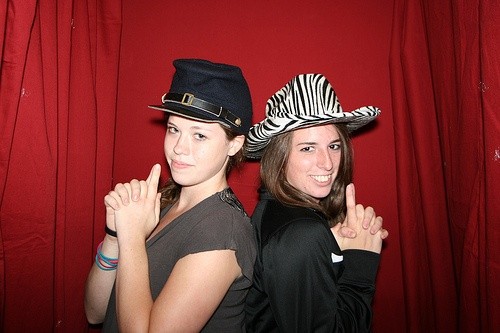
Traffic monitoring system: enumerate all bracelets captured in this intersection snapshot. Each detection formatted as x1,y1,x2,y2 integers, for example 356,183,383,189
96,241,118,271
104,226,117,238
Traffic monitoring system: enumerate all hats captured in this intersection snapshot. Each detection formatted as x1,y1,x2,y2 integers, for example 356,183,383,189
147,59,252,137
242,73,381,158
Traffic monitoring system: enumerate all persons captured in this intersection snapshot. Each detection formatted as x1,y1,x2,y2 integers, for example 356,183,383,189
83,58,258,333
247,73,389,333
